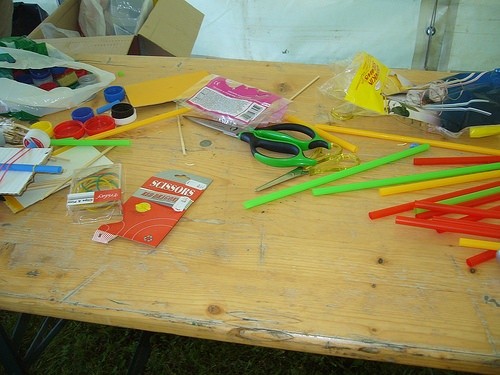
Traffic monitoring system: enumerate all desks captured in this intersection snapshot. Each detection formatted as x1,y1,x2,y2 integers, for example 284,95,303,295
0,51,500,373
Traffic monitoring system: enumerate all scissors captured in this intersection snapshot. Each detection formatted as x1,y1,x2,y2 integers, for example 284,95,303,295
182,113,334,166
253,142,359,196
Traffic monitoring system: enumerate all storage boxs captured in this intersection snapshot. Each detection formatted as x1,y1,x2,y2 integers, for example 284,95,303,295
27,1,205,55
